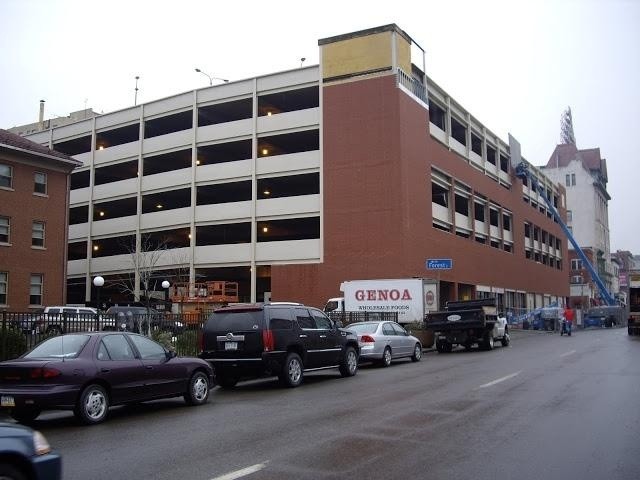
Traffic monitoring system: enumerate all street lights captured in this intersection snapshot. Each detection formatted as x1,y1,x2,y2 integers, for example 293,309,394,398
130,54,308,108
162,281,170,313
93,276,105,330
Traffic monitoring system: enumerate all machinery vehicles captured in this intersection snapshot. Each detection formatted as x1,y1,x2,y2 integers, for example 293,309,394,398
514,158,625,328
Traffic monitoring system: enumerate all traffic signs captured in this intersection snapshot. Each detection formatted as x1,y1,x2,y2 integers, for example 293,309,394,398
407,225,454,269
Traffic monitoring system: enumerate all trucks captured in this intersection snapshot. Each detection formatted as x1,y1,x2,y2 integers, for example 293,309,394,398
626,276,639,337
423,298,511,353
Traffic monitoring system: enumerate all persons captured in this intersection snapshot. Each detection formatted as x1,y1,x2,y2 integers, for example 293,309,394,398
563,305,575,336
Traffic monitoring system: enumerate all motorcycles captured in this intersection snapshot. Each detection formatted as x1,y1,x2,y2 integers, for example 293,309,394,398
559,317,572,336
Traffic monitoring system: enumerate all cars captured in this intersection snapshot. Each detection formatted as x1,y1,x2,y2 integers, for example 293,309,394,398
18,305,182,339
0,331,217,429
338,320,423,368
0,416,64,479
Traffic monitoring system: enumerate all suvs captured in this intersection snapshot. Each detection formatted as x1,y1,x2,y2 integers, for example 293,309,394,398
197,301,362,389
